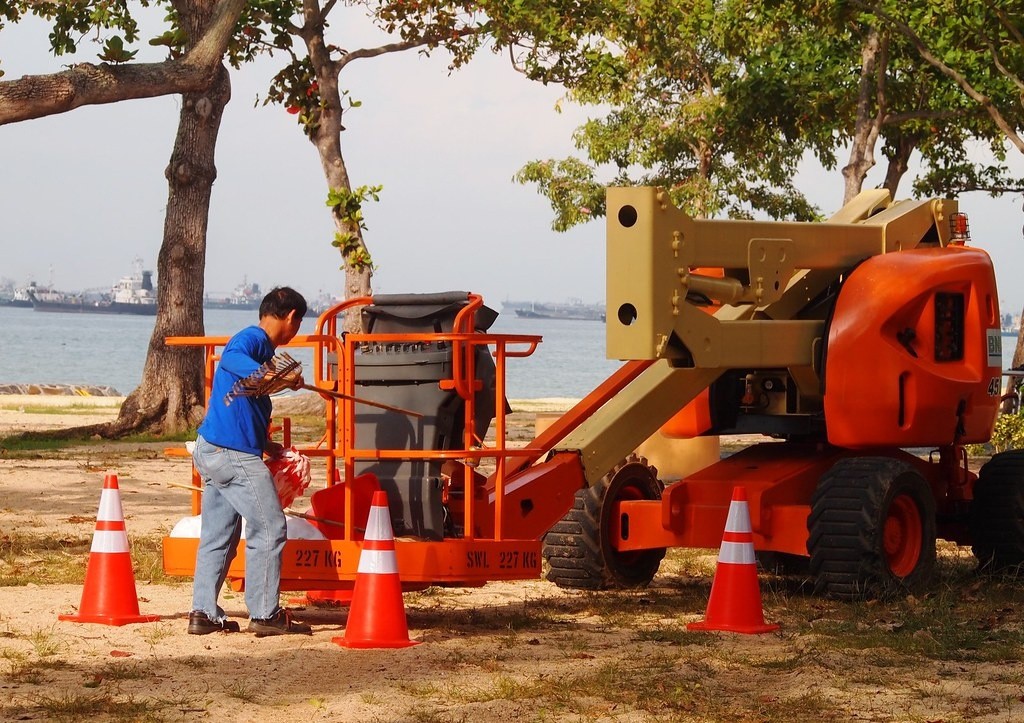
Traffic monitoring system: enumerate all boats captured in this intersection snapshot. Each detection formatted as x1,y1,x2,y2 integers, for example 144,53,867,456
0,251,349,319
500,298,606,322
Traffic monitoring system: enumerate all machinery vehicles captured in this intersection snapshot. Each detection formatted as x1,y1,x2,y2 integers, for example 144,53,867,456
158,186,1024,596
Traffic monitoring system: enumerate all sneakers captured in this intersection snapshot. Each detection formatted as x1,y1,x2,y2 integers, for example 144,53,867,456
248,606,312,636
188,611,239,635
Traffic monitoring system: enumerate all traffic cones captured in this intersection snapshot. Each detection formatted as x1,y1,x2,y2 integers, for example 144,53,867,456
57,474,158,626
686,483,780,633
328,489,421,648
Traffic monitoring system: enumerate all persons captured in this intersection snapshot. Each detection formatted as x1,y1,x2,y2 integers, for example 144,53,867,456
186,286,313,636
